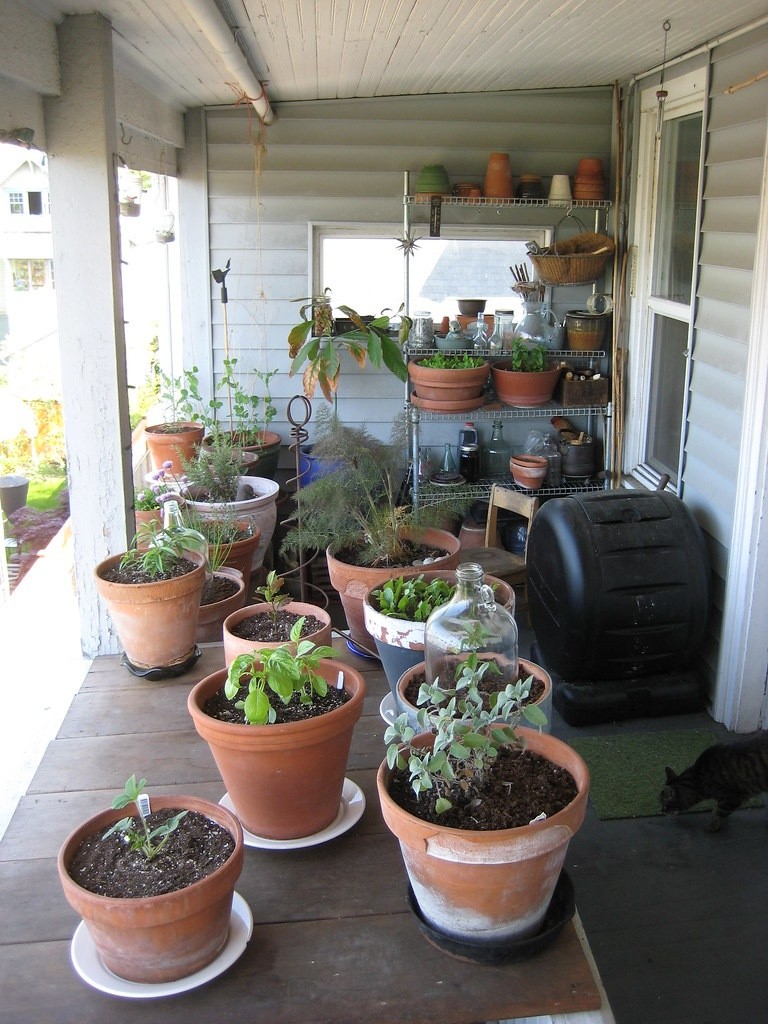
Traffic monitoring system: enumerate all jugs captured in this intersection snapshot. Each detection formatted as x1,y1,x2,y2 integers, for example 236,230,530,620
514,303,558,348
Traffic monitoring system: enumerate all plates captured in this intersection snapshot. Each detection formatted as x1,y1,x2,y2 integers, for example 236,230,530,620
429,479,466,486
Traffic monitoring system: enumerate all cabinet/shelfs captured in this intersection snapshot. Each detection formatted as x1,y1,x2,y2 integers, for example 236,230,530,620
402,170,623,528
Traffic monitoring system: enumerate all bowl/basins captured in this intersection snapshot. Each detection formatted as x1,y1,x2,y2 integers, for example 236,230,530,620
435,335,472,353
509,454,548,489
457,299,487,316
415,164,450,193
573,158,605,205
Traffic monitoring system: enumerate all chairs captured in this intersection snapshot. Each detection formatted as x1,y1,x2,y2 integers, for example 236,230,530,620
458,482,540,629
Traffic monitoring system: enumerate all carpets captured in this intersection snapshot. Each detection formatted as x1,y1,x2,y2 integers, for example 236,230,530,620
569,728,765,821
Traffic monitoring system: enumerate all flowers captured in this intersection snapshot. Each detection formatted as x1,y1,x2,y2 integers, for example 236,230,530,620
136,460,242,579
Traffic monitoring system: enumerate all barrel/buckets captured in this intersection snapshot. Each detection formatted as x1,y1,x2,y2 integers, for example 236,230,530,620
299,443,351,487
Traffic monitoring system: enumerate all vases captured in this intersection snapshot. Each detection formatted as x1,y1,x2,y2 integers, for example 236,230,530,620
416,151,608,207
191,572,245,644
458,516,506,551
509,454,549,489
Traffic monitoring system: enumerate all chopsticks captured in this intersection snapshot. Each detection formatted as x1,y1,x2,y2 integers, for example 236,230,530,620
510,262,529,282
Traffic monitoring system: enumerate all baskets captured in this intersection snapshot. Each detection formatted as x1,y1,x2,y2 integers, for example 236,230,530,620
526,215,613,283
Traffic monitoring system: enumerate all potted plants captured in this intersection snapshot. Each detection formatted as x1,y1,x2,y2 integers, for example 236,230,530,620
142,351,206,478
190,612,364,840
55,778,245,985
407,350,491,413
91,518,207,671
276,395,492,655
172,355,283,480
168,419,279,570
373,623,588,945
491,335,560,409
287,287,414,489
134,482,174,548
360,565,514,715
219,568,333,670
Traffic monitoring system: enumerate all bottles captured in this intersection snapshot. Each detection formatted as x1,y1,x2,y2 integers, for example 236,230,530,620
516,175,545,204
408,312,435,351
494,307,514,335
424,448,436,476
424,562,518,692
439,443,457,479
418,447,427,482
487,317,502,353
460,444,478,481
452,183,481,203
482,420,512,479
458,422,477,473
473,312,487,330
546,444,561,487
472,322,487,352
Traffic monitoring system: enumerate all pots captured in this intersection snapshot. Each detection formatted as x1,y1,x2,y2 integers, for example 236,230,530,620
559,443,595,474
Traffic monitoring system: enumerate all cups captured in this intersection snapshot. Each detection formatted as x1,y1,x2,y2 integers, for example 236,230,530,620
483,153,512,203
548,175,572,204
566,310,606,356
